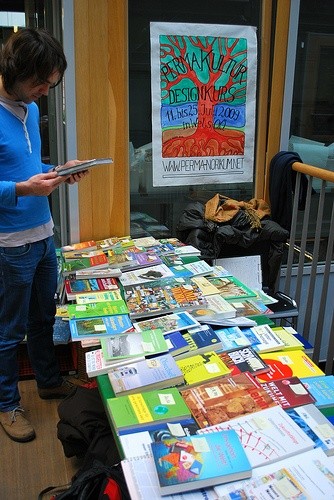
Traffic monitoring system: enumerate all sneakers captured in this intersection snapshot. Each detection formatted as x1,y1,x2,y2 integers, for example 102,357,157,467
0,407,35,442
38,379,75,399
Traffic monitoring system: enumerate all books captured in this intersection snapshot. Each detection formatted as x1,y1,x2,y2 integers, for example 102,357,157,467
54,236,334,500
57,158,112,175
130,212,170,238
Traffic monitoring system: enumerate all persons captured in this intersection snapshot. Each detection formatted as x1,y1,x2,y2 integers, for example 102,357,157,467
0,27,97,442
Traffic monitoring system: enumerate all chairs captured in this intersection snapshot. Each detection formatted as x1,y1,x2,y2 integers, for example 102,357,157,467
177,192,299,330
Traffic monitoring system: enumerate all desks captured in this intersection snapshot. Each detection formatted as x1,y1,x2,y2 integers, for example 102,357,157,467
56,238,334,500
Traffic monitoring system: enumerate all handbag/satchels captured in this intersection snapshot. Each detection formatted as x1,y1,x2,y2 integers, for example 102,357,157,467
38,465,131,500
